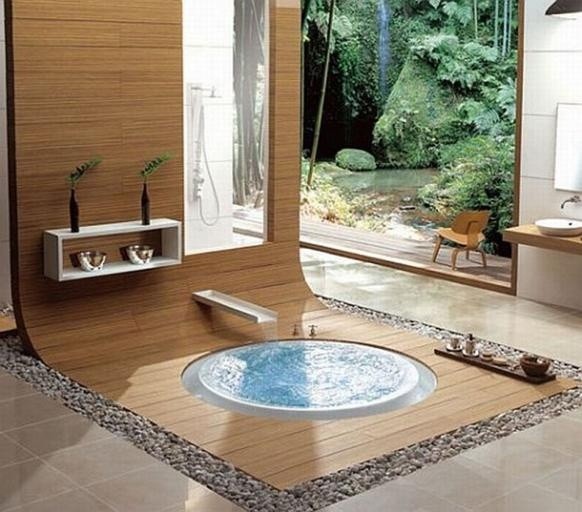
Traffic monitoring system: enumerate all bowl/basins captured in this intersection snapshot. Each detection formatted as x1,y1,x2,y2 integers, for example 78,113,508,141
126,244,154,265
520,357,550,377
76,251,107,272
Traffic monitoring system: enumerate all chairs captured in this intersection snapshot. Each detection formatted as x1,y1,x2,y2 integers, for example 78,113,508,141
433,210,493,270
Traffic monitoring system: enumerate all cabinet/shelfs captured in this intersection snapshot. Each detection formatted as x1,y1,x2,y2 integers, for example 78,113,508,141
43,217,182,282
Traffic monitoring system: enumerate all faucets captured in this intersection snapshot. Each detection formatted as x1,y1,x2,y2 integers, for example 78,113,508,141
561,196,581,209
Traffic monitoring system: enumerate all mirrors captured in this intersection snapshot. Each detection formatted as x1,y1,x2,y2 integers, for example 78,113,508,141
554,103,582,193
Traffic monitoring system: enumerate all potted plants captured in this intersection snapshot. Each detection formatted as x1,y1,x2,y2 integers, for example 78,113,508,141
137,152,174,224
64,155,103,232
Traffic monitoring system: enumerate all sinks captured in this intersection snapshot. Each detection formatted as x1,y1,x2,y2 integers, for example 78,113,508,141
536,218,582,236
